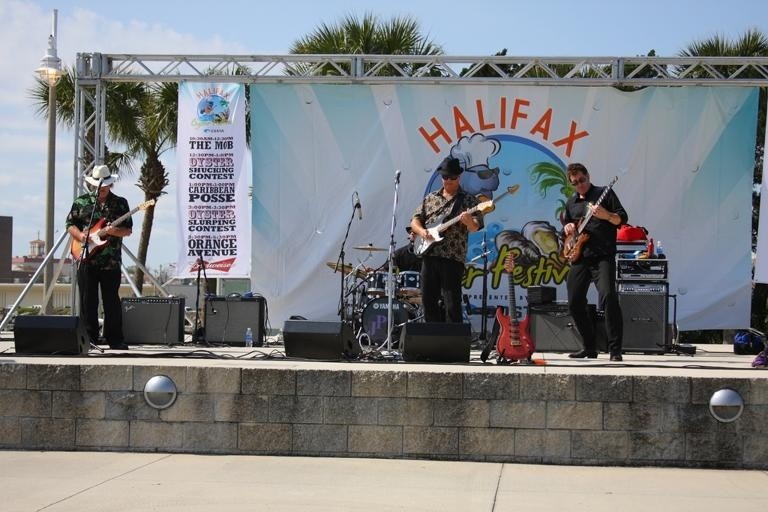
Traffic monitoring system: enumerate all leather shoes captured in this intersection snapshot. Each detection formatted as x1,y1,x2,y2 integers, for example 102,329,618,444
610,353,622,361
569,349,598,358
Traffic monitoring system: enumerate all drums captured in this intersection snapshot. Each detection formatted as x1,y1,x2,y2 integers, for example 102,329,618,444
360,296,409,346
396,271,421,295
368,272,389,296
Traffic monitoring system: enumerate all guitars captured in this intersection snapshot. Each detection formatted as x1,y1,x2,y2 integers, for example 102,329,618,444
563,175,618,261
71,199,155,262
414,200,494,258
496,252,535,358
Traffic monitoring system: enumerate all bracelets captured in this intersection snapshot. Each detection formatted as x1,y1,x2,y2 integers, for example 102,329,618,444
608,212,612,220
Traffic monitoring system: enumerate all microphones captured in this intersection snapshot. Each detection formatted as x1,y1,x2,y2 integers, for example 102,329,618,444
396,170,402,185
482,234,485,248
94,177,105,196
355,197,364,220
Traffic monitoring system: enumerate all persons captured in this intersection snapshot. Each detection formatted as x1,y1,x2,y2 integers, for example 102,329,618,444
384,222,425,272
563,162,629,361
65,164,134,352
410,157,486,325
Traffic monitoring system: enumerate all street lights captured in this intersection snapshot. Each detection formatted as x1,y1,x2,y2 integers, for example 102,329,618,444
35,6,65,311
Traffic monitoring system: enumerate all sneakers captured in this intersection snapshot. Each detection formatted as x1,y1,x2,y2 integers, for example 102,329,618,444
110,343,128,350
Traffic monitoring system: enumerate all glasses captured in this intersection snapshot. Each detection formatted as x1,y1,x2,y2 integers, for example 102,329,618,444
442,175,460,180
570,177,586,185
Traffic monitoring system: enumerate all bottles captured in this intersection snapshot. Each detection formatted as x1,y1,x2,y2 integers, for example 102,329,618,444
647,237,654,259
655,241,664,258
245,327,252,347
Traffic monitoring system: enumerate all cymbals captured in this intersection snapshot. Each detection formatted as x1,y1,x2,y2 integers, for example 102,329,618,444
352,246,388,251
327,262,367,277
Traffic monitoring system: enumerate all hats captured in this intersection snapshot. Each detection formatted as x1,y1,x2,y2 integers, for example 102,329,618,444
437,158,464,176
85,165,119,187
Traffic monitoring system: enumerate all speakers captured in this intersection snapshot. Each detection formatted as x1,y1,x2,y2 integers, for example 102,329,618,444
616,280,669,355
526,301,596,352
204,297,264,346
14,316,90,355
120,297,184,345
283,320,362,362
398,323,472,363
